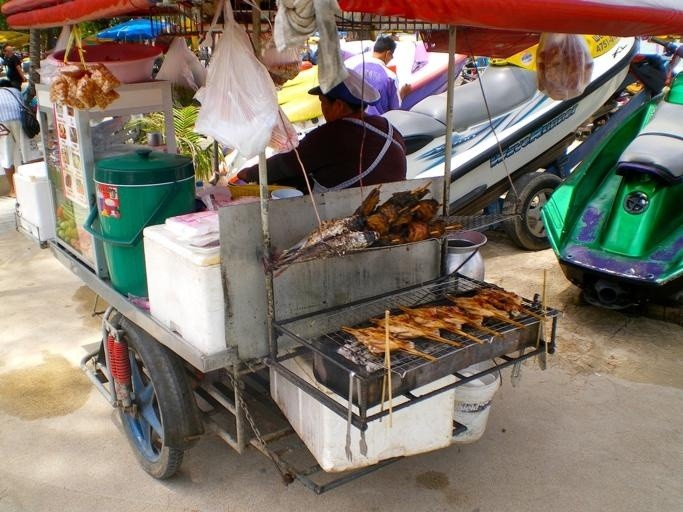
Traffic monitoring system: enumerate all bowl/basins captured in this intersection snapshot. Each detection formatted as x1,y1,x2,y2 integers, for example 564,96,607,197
48,43,163,84
271,189,303,200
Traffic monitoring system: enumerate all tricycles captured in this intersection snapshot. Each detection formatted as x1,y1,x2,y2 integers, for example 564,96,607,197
16,114,561,495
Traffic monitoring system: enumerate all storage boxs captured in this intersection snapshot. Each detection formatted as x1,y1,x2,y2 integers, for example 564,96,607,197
143,196,279,355
268,344,454,471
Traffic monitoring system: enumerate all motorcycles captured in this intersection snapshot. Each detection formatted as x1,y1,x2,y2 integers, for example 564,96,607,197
540,38,683,311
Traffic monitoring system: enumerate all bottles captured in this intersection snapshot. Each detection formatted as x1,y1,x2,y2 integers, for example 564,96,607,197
195,181,206,199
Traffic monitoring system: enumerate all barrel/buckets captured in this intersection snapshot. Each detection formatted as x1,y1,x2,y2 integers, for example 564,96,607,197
455,358,502,444
82,146,197,297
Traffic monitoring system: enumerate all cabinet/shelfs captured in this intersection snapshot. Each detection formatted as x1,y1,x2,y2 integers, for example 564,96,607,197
34,79,178,280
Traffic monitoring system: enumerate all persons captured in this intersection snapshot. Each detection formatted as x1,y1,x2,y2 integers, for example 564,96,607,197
0,79,43,198
4,45,26,91
353,36,401,116
237,68,406,195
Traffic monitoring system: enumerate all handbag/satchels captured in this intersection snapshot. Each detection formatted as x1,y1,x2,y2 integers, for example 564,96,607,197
20,106,40,139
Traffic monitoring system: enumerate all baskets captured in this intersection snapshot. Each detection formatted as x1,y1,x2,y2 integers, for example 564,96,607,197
226,184,295,201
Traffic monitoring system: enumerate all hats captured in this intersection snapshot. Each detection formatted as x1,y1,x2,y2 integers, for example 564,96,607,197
308,84,382,106
